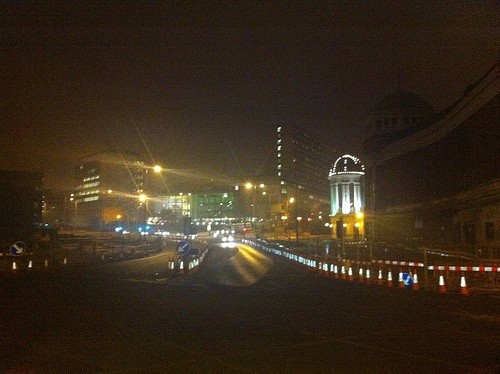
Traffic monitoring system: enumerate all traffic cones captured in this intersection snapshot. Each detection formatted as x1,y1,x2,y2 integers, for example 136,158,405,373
438,270,446,294
242,238,339,278
363,269,371,285
339,266,347,279
358,268,364,283
397,267,404,289
167,249,210,276
347,268,354,281
63,258,67,270
12,262,17,275
459,272,470,296
377,270,383,286
387,266,393,287
43,259,49,272
28,261,32,273
411,269,420,292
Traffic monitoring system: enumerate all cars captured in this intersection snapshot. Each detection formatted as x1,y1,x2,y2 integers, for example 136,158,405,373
216,234,233,243
